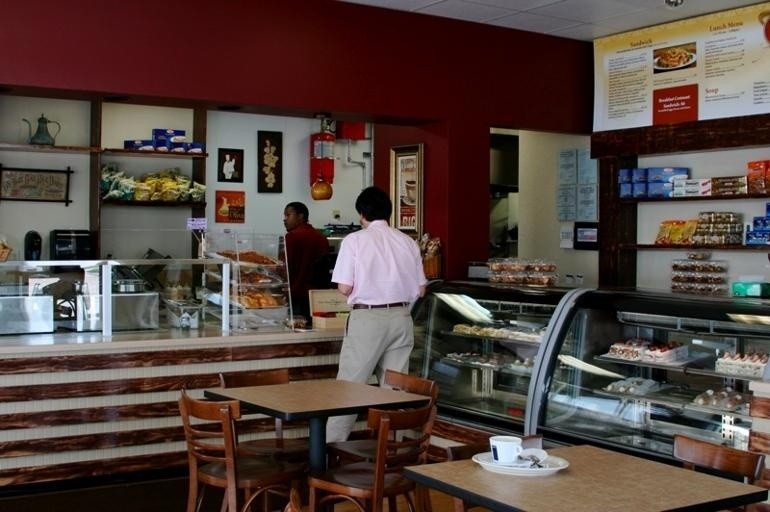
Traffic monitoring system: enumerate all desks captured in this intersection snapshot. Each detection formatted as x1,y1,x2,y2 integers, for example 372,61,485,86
397,441,768,512
198,372,438,512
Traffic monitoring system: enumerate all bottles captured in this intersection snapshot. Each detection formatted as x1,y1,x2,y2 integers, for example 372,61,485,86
566,271,575,283
575,270,585,286
165,281,192,301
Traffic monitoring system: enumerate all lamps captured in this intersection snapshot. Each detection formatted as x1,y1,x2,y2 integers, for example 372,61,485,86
311,111,333,202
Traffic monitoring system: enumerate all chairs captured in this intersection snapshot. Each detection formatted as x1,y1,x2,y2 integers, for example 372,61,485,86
173,388,295,512
219,366,313,512
303,403,438,512
670,429,766,512
320,367,442,512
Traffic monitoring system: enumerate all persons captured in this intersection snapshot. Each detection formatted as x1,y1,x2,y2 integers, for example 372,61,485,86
278,202,330,316
324,184,429,471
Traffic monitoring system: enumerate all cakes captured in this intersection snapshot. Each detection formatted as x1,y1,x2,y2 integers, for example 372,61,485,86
609,339,689,362
715,351,769,378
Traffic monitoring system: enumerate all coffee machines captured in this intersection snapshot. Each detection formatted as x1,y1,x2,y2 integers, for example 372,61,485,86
47,228,94,275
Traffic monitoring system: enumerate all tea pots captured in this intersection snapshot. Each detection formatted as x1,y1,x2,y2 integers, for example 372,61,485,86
223,154,236,179
21,112,63,147
218,197,232,217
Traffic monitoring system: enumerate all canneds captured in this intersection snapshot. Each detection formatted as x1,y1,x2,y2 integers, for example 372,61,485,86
694,212,743,245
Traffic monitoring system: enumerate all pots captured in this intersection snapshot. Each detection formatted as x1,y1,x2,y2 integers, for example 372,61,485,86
110,276,149,293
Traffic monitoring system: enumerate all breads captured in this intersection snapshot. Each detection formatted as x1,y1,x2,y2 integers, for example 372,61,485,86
222,250,278,306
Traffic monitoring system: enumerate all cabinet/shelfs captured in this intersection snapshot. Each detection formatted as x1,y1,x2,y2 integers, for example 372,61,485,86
90,143,208,213
585,112,769,302
194,228,298,337
427,293,585,420
564,316,770,482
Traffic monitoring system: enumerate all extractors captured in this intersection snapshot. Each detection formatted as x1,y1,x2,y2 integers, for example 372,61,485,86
490,139,519,200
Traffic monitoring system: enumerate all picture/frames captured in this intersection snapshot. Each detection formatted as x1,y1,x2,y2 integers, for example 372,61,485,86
388,143,425,243
2,164,75,208
217,148,243,183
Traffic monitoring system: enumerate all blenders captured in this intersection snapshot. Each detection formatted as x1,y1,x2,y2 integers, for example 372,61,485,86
23,229,47,284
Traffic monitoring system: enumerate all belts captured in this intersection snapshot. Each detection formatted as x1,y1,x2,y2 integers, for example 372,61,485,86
353,302,409,309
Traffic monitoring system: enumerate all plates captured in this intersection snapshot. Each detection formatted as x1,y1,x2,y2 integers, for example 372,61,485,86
651,51,699,71
519,447,548,467
471,449,570,478
402,197,417,206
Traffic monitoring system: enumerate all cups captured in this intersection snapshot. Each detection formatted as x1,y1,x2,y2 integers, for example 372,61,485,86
488,434,523,466
406,181,417,202
